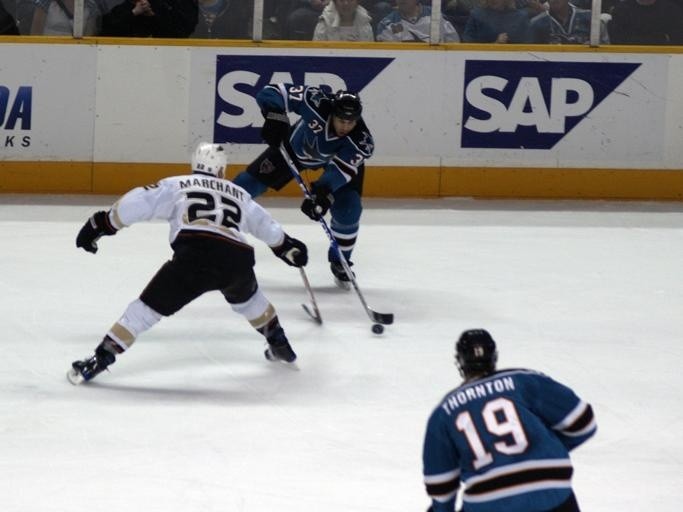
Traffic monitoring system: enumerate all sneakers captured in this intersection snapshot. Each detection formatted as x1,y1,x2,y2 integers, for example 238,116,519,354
331,261,354,281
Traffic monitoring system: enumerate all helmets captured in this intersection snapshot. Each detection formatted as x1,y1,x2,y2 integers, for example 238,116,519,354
192,143,227,179
333,91,362,119
454,330,496,379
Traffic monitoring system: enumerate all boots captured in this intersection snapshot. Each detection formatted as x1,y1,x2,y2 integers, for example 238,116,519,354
73,357,102,381
265,328,295,362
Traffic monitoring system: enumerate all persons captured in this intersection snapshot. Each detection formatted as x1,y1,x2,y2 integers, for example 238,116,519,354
423,332,597,512
66,141,309,381
0,0,683,46
229,82,374,282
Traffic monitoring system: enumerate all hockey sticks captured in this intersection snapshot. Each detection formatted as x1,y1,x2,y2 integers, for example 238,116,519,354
279,143,393,324
293,251,322,324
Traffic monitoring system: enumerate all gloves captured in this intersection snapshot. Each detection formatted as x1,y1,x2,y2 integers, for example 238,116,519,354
271,234,307,267
261,112,291,148
301,184,335,220
76,211,117,253
